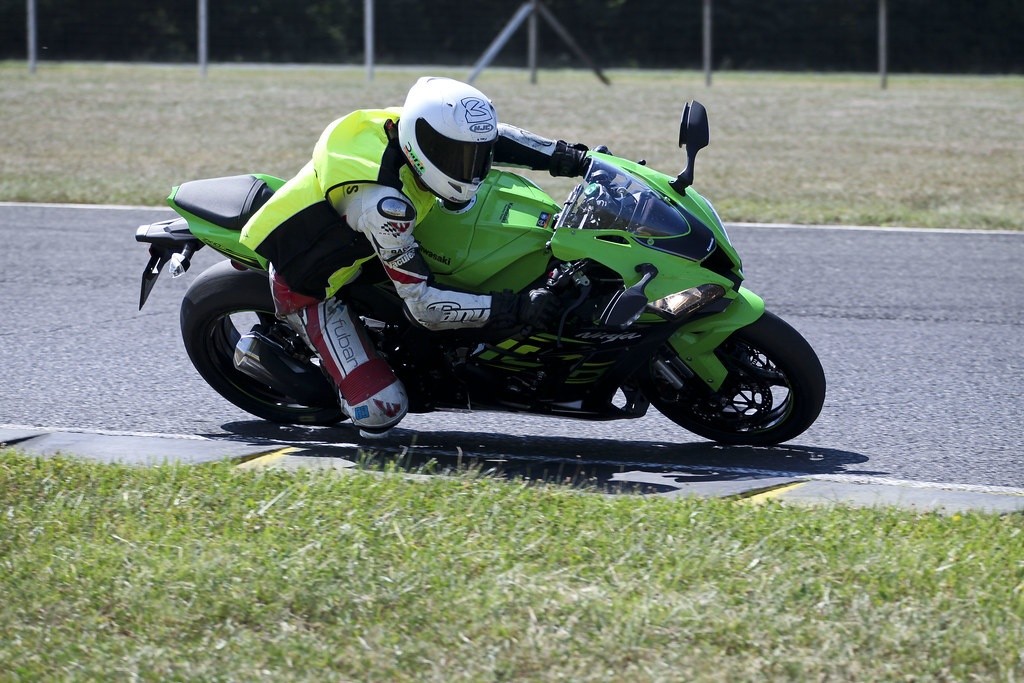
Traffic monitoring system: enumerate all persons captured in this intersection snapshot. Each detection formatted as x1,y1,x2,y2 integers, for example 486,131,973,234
240,76,621,440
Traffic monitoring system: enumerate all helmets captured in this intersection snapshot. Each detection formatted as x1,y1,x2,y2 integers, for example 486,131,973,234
396,76,496,205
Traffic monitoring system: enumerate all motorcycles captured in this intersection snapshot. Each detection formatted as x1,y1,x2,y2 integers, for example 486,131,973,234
132,99,826,448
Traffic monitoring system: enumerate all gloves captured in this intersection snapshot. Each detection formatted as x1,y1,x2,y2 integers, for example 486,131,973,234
489,288,557,322
547,140,615,183
